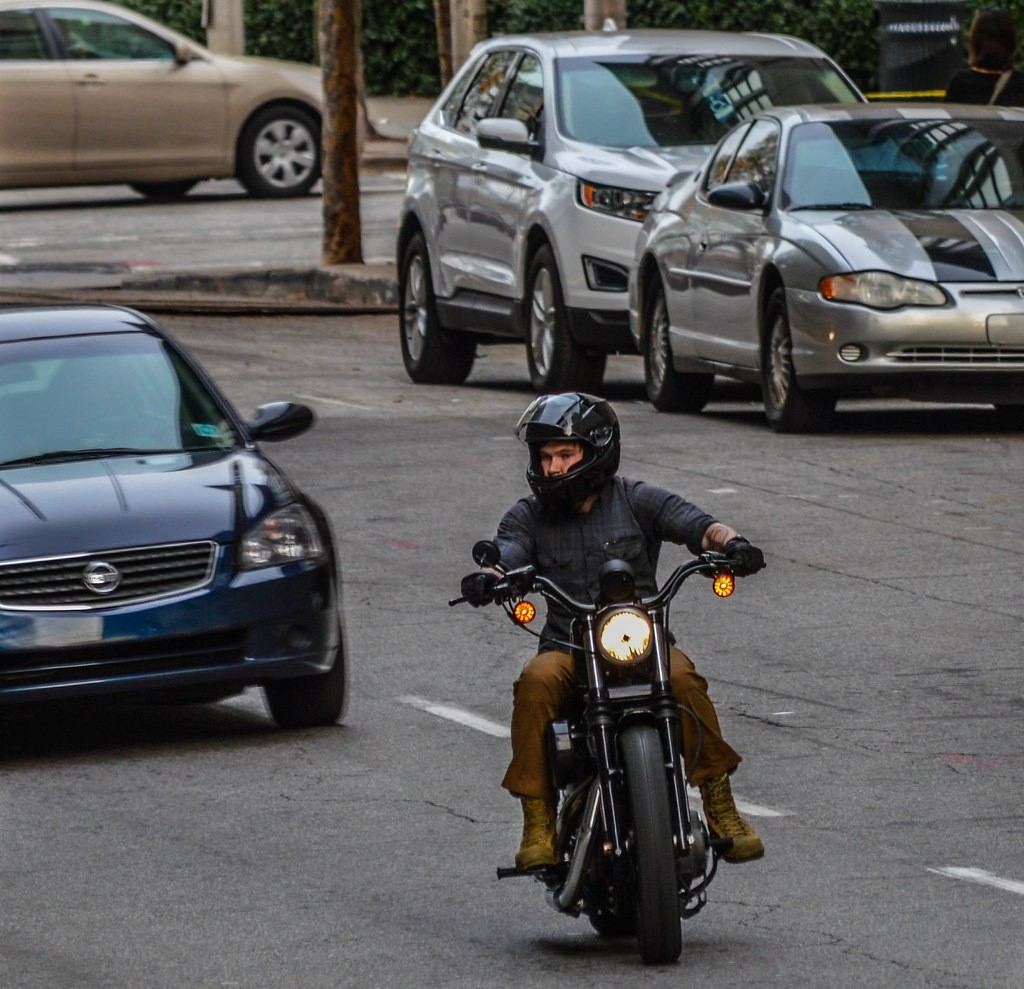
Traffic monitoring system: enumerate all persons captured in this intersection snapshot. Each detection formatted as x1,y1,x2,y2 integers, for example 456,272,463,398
941,8,1024,106
460,389,766,876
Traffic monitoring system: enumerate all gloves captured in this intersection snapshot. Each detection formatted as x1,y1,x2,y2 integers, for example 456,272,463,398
721,537,764,578
460,571,504,608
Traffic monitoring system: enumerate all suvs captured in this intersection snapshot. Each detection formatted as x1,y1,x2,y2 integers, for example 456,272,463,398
392,25,872,400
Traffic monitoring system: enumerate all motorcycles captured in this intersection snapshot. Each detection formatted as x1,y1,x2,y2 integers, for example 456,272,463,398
444,538,767,967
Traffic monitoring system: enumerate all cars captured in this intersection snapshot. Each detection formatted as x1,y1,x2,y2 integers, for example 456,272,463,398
0,1,366,205
0,304,351,733
628,100,1024,434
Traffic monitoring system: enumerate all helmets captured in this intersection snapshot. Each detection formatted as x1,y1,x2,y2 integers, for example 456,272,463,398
515,392,620,506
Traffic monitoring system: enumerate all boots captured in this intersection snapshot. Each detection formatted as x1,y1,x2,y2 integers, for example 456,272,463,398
515,796,563,869
700,773,764,864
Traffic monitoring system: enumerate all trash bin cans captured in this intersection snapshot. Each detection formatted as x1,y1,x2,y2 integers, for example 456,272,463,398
877,21,963,102
